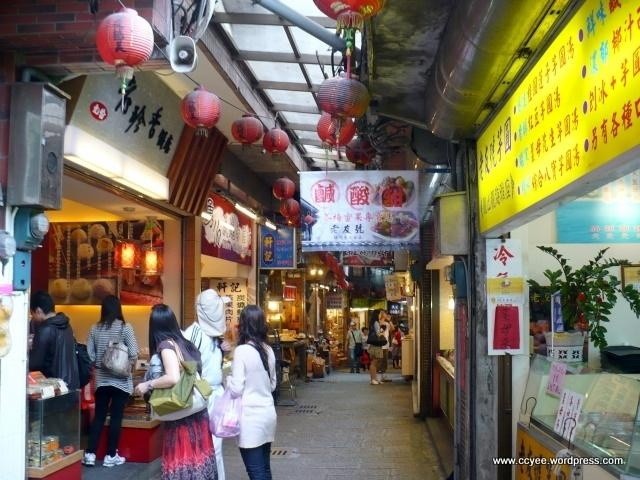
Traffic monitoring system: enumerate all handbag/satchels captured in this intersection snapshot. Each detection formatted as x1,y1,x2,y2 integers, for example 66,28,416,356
74,338,92,389
146,337,197,417
359,350,370,365
354,341,363,356
102,321,133,378
367,319,388,347
391,336,399,345
206,383,243,439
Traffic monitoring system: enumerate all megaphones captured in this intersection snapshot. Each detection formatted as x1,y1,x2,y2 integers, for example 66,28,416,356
169,35,198,73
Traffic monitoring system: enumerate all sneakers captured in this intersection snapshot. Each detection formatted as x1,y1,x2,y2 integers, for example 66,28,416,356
369,379,384,386
350,367,360,373
81,449,97,466
102,449,127,467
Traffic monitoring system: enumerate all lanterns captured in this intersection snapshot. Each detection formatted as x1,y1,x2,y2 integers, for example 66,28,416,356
232,112,263,152
95,8,155,112
313,0,382,37
316,76,370,150
181,84,221,138
272,178,299,217
263,128,290,153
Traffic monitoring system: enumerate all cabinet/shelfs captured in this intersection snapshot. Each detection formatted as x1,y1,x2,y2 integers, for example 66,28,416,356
517,352,639,478
82,374,161,464
27,392,85,480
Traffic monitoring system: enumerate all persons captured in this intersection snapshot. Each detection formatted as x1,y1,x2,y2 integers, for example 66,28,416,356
81,294,139,467
344,308,404,384
134,304,218,480
226,304,278,480
29,291,81,448
181,288,232,480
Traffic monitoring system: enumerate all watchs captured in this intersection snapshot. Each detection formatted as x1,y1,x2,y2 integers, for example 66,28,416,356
148,381,154,391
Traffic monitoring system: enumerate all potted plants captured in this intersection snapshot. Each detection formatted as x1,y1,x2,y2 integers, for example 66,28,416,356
525,244,640,364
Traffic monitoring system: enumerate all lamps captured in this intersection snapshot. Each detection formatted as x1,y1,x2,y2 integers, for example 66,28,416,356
109,208,138,278
139,216,168,277
264,296,286,320
282,284,298,303
